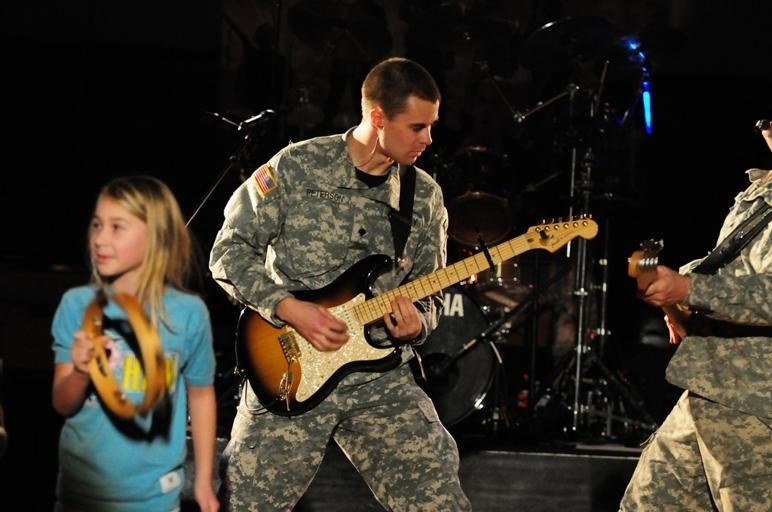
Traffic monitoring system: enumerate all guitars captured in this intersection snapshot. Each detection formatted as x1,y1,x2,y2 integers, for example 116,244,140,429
627,237,693,340
237,212,600,416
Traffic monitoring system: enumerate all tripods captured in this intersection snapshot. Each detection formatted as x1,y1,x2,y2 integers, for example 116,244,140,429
460,60,659,454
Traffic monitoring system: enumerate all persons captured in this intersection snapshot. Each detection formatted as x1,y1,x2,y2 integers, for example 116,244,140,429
618,118,772,512
51,176,221,512
208,56,473,512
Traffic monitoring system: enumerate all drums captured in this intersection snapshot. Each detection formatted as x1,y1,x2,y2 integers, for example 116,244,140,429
483,260,598,351
437,145,515,248
445,245,520,293
409,284,498,428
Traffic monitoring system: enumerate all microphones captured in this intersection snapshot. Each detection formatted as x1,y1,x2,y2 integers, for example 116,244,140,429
239,109,277,131
